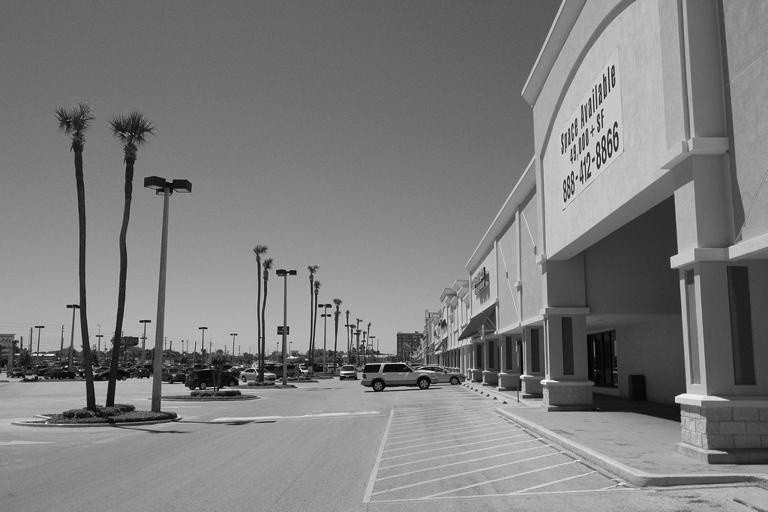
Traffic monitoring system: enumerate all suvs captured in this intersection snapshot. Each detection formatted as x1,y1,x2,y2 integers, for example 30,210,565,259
184,369,239,388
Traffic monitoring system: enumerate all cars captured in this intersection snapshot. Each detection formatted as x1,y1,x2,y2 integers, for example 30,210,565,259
340,364,357,380
360,362,465,392
7,360,309,390
240,367,276,382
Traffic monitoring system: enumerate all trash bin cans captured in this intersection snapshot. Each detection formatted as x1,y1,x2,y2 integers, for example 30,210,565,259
628,375,646,401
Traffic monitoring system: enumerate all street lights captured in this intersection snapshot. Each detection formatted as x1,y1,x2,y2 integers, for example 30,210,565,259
66,304,80,369
230,334,237,355
198,326,208,351
144,176,192,412
139,320,151,359
276,269,297,385
35,326,44,356
318,303,332,372
